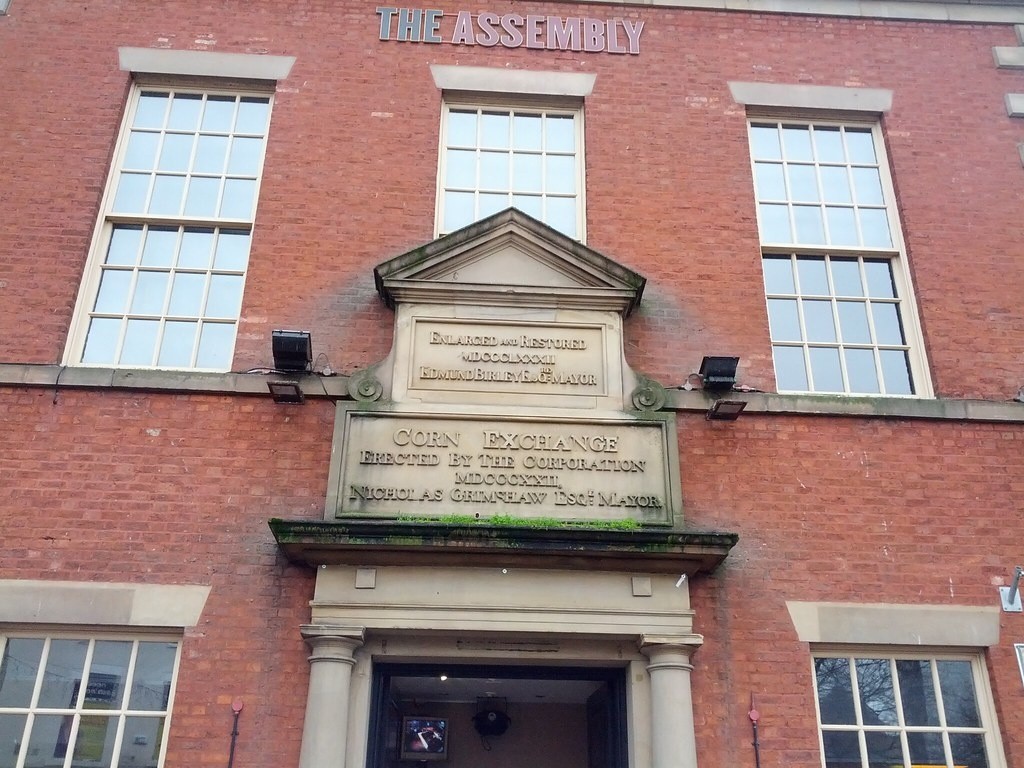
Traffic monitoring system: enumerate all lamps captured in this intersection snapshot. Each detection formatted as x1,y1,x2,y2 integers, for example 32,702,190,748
272,328,315,376
706,400,748,421
266,380,306,406
678,357,741,391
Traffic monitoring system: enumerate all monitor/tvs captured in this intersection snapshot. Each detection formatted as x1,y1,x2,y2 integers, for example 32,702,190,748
400,716,449,760
